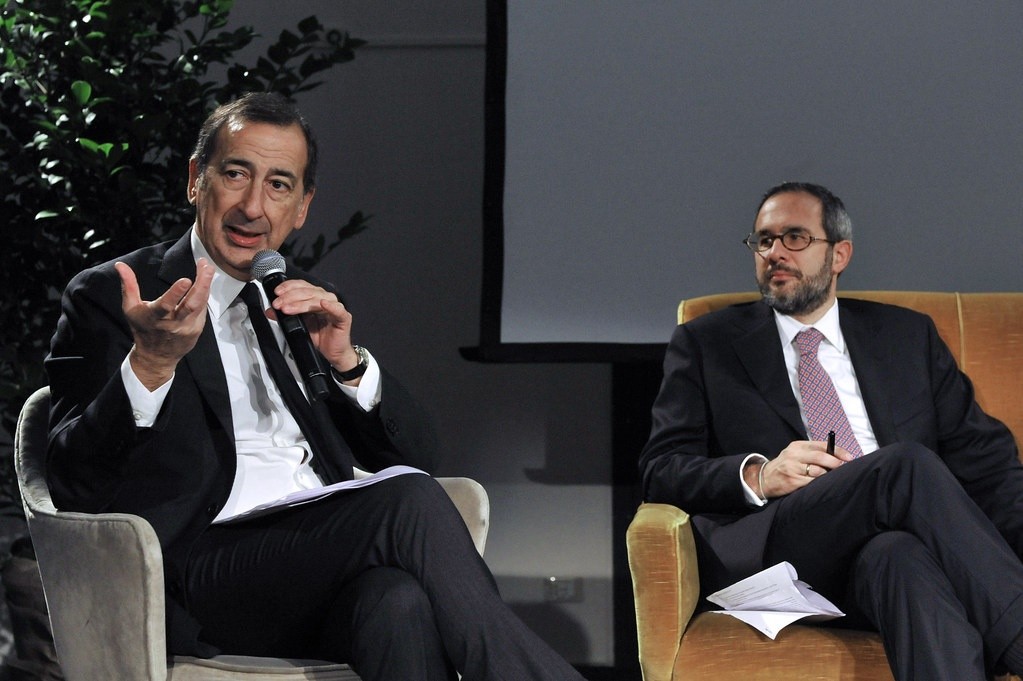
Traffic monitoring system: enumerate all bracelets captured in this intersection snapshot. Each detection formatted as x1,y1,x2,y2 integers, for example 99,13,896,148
758,459,771,503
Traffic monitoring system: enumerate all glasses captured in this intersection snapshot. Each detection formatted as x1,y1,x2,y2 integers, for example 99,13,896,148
745,231,836,250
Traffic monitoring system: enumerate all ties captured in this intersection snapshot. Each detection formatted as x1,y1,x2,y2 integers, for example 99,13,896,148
239,283,355,487
795,327,864,465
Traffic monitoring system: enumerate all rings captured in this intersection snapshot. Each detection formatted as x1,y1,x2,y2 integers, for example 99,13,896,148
805,463,811,476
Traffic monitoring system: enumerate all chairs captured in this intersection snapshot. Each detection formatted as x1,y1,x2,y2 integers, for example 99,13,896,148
624,289,1023,681
12,382,492,681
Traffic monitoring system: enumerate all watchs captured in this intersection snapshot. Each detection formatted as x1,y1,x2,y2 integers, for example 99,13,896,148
330,344,370,387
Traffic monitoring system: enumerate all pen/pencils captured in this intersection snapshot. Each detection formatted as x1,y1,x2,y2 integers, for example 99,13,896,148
827,431,837,473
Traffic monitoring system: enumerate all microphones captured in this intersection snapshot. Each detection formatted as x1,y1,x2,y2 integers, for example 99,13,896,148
251,249,331,403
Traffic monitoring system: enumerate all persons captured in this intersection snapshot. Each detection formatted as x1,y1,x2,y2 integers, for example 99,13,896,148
635,179,1023,681
39,82,596,681
